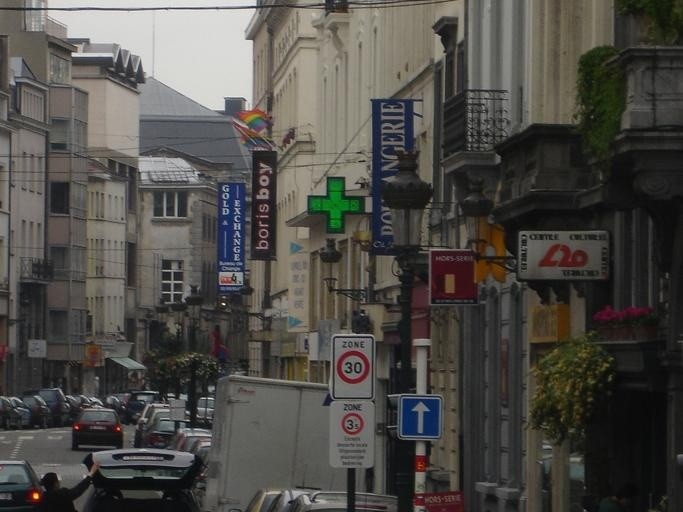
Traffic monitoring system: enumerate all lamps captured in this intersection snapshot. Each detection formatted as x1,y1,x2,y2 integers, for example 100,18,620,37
458,167,572,305
322,276,367,303
239,278,271,322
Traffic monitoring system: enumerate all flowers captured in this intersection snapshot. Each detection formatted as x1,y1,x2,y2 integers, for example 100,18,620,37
524,331,616,447
593,303,659,327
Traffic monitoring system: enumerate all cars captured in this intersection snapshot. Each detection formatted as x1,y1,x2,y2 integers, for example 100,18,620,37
77,445,202,511
227,484,398,512
0,386,214,482
0,458,46,511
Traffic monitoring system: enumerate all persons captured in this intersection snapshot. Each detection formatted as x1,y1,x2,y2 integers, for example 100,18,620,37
41,461,100,512
598,480,638,512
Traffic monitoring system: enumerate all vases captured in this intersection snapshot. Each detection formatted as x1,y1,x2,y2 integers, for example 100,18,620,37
580,377,609,400
595,326,657,340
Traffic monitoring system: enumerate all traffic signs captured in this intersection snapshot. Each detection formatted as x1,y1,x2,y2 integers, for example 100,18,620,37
332,333,374,400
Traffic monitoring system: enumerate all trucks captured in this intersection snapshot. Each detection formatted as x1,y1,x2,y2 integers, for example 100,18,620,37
204,374,366,512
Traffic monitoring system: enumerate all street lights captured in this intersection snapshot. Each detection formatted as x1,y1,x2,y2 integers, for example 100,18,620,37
183,283,203,427
380,147,436,512
169,292,186,399
154,295,169,397
